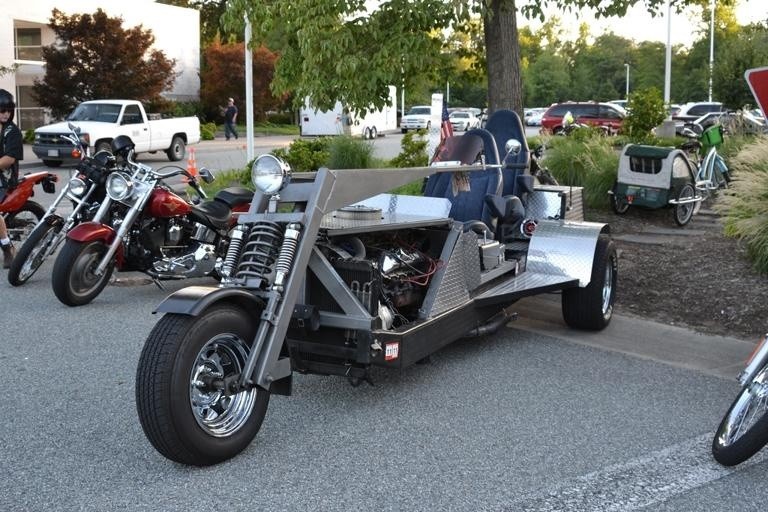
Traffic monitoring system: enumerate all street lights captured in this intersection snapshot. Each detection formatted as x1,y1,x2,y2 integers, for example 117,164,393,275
622,63,630,96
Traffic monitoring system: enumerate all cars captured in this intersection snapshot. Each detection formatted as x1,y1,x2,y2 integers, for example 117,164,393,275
524,107,550,126
447,106,488,133
662,101,768,137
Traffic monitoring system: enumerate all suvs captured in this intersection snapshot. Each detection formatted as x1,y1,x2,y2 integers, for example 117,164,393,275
399,104,431,135
538,98,628,137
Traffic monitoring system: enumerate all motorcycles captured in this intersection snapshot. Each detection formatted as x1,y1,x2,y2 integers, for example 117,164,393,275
131,101,623,469
706,328,767,469
8,116,205,287
0,165,55,269
47,130,255,312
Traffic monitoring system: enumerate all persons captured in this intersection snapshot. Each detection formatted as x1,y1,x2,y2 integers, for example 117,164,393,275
0,89,24,269
220,97,239,141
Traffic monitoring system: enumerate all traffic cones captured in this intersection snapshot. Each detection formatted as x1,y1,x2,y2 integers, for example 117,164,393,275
180,143,198,182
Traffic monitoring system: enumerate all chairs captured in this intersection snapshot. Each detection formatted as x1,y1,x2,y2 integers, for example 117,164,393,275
422,127,503,244
483,108,532,201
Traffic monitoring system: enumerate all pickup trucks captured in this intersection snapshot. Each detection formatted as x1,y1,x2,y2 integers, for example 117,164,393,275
28,96,204,173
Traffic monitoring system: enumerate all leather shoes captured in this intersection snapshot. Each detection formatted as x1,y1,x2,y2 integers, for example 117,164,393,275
1,242,16,268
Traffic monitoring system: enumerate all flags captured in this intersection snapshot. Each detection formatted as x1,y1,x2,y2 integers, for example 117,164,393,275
440,102,453,140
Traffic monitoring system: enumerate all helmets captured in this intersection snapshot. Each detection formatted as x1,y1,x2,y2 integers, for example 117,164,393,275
111,135,134,153
0,89,14,109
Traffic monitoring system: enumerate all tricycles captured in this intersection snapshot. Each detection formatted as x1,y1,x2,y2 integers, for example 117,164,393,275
607,120,734,228
